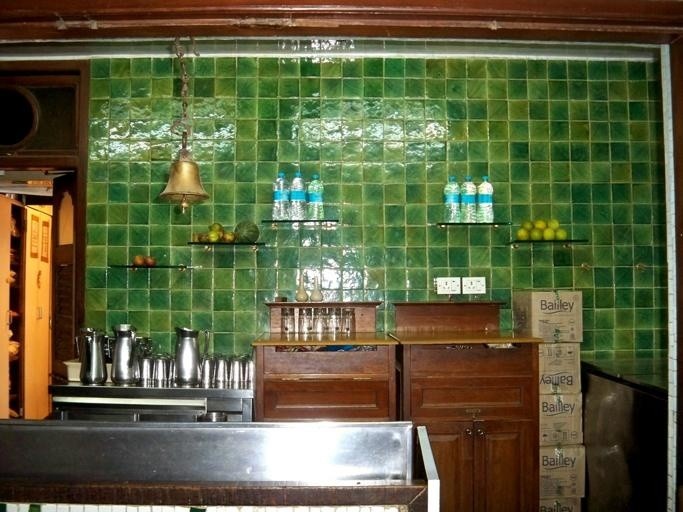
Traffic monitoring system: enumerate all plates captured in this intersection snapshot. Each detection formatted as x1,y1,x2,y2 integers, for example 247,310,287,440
0,194,53,420
389,302,543,512
109,219,588,269
251,302,398,421
48,385,252,423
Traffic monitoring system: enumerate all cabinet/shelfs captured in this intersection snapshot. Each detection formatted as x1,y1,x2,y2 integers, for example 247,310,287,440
295,274,323,302
444,175,494,223
281,307,356,334
272,172,324,221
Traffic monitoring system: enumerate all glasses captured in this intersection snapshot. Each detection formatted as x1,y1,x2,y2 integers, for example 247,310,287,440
158,34,210,215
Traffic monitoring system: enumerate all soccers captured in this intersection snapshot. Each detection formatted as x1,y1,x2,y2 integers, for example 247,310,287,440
144,256,156,265
133,255,144,266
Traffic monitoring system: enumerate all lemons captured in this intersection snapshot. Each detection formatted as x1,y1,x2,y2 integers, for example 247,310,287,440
111,324,140,383
175,326,210,383
80,327,107,384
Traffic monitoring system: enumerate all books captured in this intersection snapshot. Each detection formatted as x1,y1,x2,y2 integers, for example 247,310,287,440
539,498,581,512
539,343,582,394
513,290,584,343
539,394,583,447
539,445,585,499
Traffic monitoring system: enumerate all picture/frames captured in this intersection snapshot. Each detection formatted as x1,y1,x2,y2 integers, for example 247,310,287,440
581,358,667,512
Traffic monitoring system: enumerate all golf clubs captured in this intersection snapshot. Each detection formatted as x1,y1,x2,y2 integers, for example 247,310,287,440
235,221,259,243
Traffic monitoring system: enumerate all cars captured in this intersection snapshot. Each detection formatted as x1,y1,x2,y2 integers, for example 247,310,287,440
140,353,177,380
200,354,253,383
